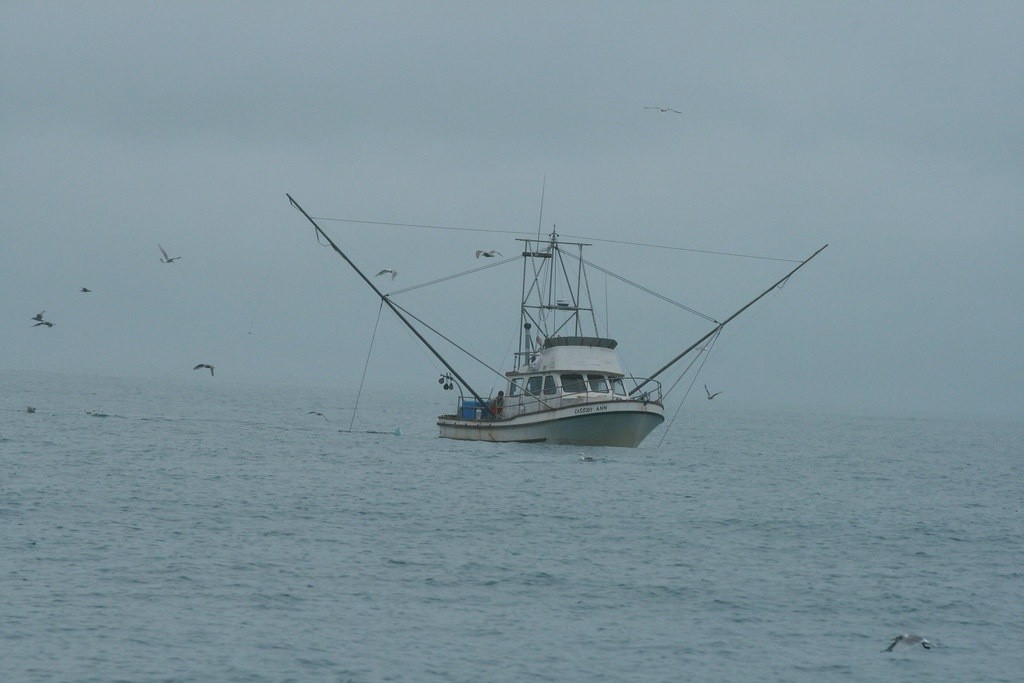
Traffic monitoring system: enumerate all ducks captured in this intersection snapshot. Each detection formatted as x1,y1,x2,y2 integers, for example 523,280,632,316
27,406,38,414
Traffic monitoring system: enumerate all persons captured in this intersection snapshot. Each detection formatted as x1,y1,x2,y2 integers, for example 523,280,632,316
491,391,504,413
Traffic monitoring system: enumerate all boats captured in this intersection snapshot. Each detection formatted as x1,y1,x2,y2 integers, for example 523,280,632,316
286,191,827,448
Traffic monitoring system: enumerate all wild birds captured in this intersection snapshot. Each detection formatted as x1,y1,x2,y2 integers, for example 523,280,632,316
704,384,722,400
643,106,682,114
158,245,181,263
79,287,92,293
32,311,56,328
879,633,934,654
305,412,330,422
375,269,398,281
476,249,503,259
193,363,214,376
